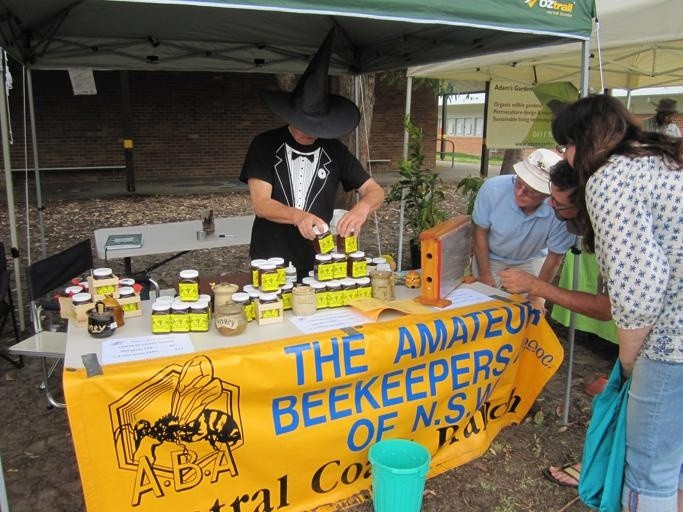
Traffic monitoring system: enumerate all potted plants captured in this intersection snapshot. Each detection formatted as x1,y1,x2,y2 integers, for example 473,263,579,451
384,115,448,269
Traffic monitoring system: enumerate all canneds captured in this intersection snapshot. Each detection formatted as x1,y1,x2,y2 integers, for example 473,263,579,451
303,224,393,310
64,267,137,323
232,257,298,323
151,270,213,335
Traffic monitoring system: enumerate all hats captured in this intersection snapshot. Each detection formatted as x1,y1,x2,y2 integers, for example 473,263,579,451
260,23,361,141
655,99,682,112
512,148,564,196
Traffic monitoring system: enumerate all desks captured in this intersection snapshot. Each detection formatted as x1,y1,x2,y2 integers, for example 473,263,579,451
93,216,255,280
7,268,540,415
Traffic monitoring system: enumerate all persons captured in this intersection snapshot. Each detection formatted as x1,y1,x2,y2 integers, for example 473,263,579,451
637,97,680,137
237,123,385,282
470,149,575,316
551,90,683,512
496,158,611,489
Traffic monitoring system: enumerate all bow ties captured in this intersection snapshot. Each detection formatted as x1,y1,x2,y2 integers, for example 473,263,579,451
291,151,314,163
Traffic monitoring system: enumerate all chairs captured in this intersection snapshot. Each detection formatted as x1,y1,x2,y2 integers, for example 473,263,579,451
24,238,160,409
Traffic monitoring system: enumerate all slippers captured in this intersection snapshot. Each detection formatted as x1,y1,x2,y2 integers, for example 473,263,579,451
541,461,582,489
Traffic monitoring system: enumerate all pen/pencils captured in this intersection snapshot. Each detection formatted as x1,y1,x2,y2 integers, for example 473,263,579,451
219,235,237,237
